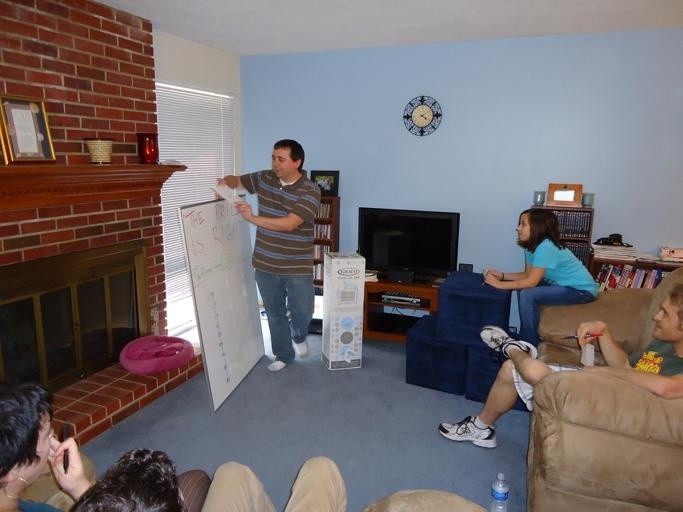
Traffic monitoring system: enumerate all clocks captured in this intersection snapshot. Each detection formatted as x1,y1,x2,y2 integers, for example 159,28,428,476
403,95,442,136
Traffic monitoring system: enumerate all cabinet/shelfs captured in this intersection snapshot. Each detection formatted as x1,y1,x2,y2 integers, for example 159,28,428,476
531,205,683,292
314,197,340,285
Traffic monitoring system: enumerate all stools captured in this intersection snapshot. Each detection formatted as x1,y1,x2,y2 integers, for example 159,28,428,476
405,270,539,413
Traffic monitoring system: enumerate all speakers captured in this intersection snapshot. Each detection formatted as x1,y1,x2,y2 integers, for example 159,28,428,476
459,264,473,273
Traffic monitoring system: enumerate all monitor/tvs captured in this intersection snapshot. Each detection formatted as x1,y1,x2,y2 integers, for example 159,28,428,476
357,207,460,283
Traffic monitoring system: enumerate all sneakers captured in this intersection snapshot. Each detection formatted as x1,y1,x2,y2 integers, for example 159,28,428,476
480,325,537,359
439,416,497,448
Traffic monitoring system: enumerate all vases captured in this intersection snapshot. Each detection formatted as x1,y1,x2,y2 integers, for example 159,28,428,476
137,132,158,164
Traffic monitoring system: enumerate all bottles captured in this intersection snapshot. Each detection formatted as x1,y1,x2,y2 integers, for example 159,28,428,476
490,472,511,512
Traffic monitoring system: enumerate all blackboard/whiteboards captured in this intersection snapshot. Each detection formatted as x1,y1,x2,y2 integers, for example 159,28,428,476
176,193,267,415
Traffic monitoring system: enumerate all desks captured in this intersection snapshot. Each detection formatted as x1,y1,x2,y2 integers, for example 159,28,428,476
363,280,440,343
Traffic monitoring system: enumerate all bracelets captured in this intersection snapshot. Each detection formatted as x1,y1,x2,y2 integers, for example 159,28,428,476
501,272,504,281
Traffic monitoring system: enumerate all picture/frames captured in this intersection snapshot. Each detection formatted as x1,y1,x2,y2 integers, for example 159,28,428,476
0,94,57,166
310,170,339,197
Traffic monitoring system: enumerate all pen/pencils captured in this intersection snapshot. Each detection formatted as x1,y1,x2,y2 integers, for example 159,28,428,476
559,332,602,340
62,421,70,473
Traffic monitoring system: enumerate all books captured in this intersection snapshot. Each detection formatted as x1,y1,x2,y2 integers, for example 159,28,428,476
551,210,591,265
595,263,671,292
313,203,331,283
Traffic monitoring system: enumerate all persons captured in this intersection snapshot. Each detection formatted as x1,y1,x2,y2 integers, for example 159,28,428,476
73,447,211,512
201,456,347,512
483,208,600,347
0,380,92,511
215,139,322,371
438,284,683,448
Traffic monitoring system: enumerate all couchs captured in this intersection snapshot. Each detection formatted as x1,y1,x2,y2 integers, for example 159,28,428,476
527,267,683,512
18,451,490,512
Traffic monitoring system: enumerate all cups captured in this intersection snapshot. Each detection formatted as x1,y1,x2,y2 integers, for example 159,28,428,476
582,192,596,208
532,190,547,206
135,131,159,164
